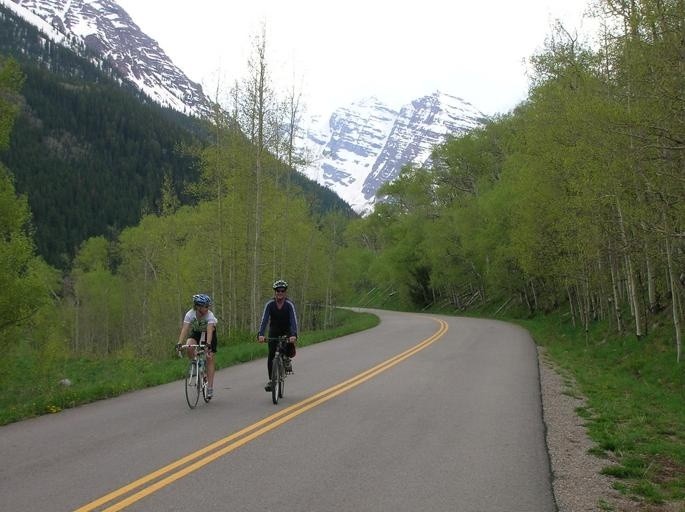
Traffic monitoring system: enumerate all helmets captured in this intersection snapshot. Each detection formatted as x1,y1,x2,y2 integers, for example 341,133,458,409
193,294,211,305
273,280,288,289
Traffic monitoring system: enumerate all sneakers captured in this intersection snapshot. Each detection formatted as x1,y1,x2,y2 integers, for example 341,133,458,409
265,381,272,392
191,360,201,367
284,360,292,370
207,389,213,397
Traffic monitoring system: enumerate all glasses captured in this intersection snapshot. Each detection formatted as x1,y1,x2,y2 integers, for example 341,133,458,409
205,306,208,308
277,291,284,293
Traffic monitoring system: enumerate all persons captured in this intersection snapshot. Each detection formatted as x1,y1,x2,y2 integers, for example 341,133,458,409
255,280,300,392
175,294,218,399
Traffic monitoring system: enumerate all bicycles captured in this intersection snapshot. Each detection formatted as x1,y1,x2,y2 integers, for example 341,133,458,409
175,344,213,408
257,335,292,403
